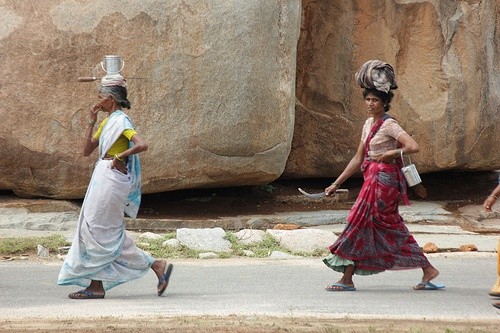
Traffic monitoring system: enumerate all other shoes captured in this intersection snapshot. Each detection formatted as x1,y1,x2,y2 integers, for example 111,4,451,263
487,277,500,296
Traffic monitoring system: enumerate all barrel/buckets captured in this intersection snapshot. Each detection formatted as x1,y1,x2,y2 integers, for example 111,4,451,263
101,55,125,75
400,150,422,187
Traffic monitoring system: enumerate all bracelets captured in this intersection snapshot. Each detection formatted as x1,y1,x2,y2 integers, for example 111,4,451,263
88,118,96,128
115,153,124,161
491,194,497,199
84,134,91,140
332,182,340,188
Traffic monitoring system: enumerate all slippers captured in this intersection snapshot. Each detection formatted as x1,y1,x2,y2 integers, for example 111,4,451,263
68,289,106,299
412,282,445,290
325,284,356,291
156,264,173,296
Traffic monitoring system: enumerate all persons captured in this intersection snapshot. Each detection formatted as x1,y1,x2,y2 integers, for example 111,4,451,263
483,183,500,309
323,88,445,291
57,74,173,299
